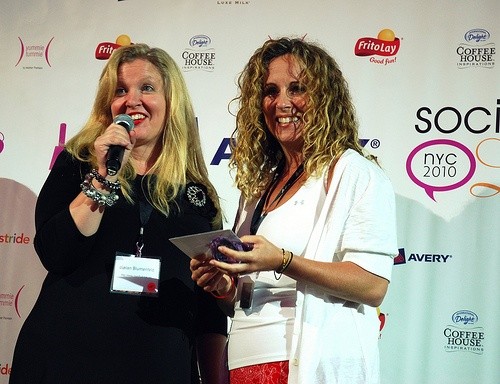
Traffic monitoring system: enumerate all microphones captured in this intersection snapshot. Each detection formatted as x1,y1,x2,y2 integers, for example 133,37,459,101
106,114,135,176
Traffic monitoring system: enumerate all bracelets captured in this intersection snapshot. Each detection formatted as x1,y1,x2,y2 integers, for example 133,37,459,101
274,248,293,280
210,274,234,298
81,167,120,207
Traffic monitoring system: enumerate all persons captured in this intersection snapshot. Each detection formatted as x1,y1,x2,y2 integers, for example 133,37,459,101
190,38,399,383
7,44,234,384
